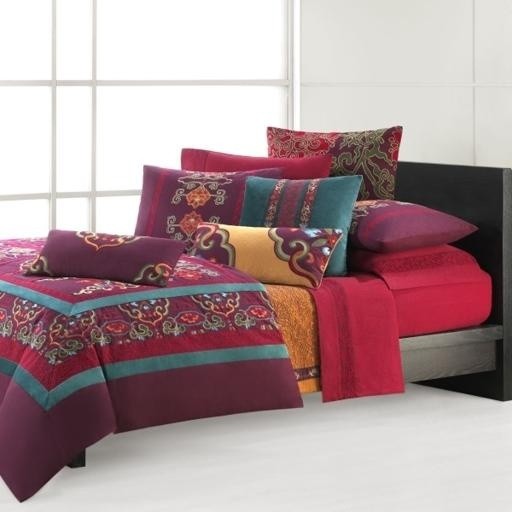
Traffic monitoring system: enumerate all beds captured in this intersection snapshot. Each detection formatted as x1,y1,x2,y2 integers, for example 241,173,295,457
0,161,512,503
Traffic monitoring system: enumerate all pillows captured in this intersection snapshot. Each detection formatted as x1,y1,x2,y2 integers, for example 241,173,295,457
189,221,343,289
239,174,362,278
22,230,188,286
360,242,484,290
134,164,285,257
351,199,476,253
266,123,403,199
180,148,332,182
